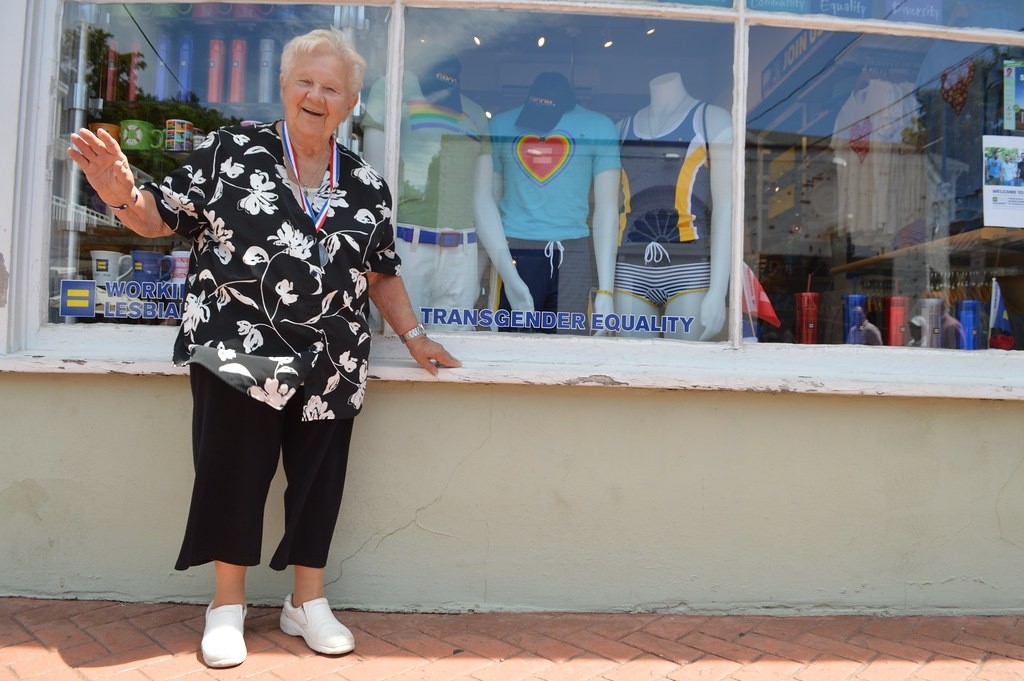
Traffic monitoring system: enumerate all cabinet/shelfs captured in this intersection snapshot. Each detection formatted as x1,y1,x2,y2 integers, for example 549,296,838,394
84,12,332,185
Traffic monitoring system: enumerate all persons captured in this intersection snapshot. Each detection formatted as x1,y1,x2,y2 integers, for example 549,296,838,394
361,47,730,342
67,28,462,668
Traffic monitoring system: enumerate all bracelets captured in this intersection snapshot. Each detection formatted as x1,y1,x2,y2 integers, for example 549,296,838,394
108,190,140,211
594,289,614,297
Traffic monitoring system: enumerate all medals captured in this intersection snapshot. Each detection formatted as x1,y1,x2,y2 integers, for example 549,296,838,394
317,242,329,268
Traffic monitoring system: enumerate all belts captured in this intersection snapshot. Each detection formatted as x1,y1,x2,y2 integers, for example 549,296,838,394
397,225,478,248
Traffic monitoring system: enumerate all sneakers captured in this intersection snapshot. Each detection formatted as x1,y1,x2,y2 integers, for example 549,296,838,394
279,593,356,655
201,600,247,669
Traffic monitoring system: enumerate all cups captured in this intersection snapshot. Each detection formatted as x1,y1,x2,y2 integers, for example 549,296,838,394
120,120,163,149
90,251,132,286
165,119,193,151
87,124,119,145
112,3,316,19
171,251,191,284
131,251,173,282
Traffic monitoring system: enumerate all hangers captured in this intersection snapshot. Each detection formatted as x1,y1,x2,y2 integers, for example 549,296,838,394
920,268,1024,318
861,278,918,312
854,53,918,96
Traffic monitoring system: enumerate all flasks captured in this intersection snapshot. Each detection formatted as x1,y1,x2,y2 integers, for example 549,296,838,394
797,292,821,344
882,297,909,346
841,295,866,343
955,300,982,349
918,298,946,348
99,38,275,105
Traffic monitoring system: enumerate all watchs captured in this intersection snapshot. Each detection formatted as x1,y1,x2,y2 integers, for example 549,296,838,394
399,323,426,344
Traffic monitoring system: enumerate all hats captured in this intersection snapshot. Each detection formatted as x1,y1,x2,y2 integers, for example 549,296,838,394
514,72,574,133
409,43,464,114
